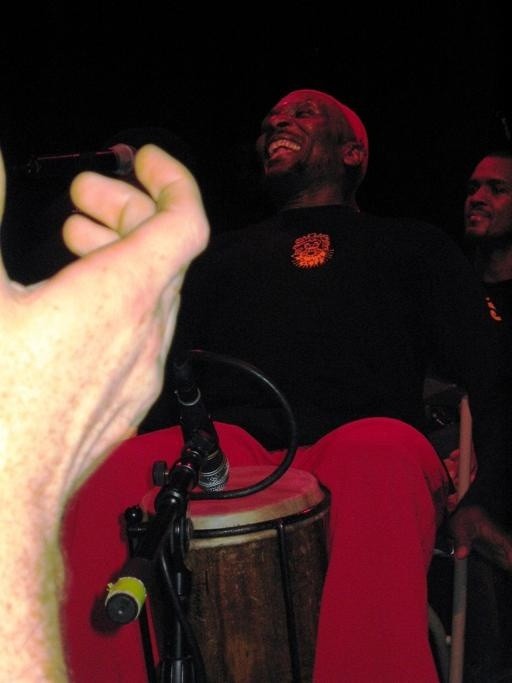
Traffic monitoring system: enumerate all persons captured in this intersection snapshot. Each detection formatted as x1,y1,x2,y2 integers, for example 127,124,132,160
59,87,509,682
0,143,211,682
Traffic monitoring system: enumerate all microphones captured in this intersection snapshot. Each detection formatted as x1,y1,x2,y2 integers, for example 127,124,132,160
27,143,137,177
172,355,231,492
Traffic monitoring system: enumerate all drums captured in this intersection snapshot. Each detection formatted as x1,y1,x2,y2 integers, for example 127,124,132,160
142,464,331,683
